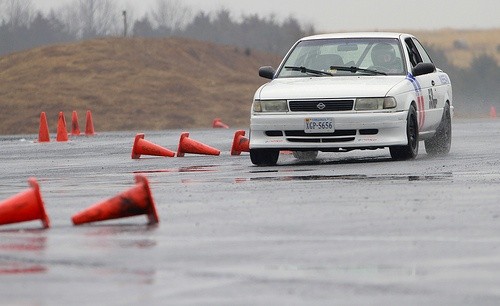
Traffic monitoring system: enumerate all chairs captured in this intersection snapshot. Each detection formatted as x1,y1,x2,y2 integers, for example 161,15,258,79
312,54,343,68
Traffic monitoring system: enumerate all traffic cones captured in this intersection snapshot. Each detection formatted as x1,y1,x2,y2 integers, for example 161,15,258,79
213,120,229,129
33,111,50,142
83,111,96,135
55,112,68,142
489,106,496,118
0,180,49,228
177,132,220,157
71,175,159,225
231,131,250,155
68,110,83,135
131,133,175,159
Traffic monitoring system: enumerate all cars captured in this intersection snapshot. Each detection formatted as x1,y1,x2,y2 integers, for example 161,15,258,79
249,32,454,165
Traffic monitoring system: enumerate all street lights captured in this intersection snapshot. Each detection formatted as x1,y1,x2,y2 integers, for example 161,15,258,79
123,10,126,36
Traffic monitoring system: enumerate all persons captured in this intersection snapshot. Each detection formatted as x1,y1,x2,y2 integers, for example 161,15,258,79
370,44,401,73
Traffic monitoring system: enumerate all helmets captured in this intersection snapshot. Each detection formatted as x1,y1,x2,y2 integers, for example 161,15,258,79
371,43,396,68
